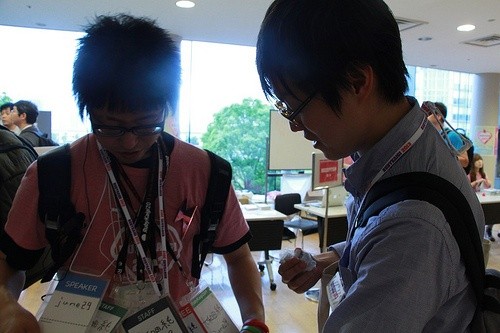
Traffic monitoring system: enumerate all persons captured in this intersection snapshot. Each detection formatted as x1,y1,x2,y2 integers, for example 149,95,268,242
0,100,60,239
255,0,486,333
427,102,496,242
0,12,269,333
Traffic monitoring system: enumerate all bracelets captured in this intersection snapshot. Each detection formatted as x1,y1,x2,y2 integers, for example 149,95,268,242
241,318,269,333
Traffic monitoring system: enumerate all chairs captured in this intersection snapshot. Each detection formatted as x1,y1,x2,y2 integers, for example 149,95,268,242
246,220,284,291
275,193,319,249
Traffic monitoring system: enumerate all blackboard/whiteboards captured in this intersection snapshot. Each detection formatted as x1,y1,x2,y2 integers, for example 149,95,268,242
268,109,334,170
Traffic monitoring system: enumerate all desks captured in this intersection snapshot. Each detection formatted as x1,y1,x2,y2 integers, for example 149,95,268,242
205,191,500,266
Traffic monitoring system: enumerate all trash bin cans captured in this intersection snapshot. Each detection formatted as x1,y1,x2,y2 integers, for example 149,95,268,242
481,238,492,268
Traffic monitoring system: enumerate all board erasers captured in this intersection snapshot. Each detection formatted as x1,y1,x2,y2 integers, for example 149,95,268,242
298,171,304,174
